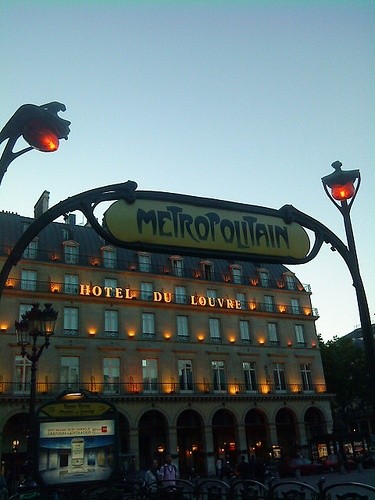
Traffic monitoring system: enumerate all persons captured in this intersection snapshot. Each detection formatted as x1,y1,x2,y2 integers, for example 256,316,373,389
159,454,178,490
236,445,369,488
143,459,161,500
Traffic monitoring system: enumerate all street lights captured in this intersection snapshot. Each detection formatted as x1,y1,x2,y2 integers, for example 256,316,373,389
320,160,360,254
0,100,71,183
15,302,59,427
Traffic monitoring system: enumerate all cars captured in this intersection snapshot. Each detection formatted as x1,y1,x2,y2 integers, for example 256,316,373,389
254,452,375,481
